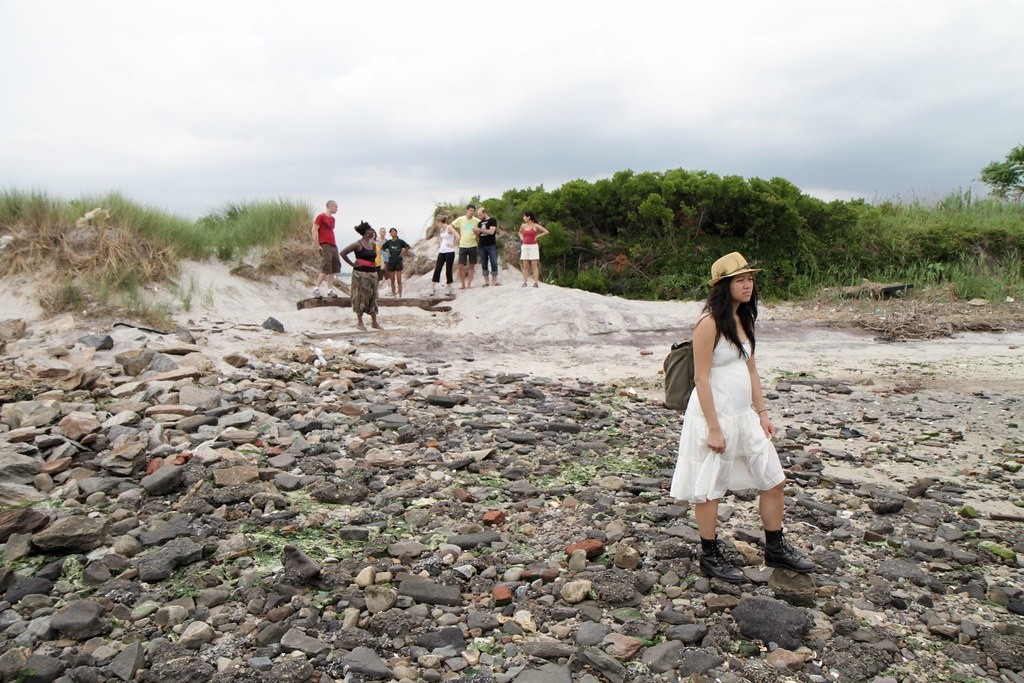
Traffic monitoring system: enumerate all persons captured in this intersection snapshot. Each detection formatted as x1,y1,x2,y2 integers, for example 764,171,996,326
518,211,549,287
429,215,460,295
311,199,342,298
449,205,481,289
339,220,384,331
375,228,394,297
473,207,503,287
669,251,817,583
370,229,383,274
381,228,411,297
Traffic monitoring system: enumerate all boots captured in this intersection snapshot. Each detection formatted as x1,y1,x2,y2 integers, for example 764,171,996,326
698,534,747,583
762,528,815,574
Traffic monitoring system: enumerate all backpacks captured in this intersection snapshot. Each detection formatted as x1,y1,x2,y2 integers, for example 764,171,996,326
664,337,696,411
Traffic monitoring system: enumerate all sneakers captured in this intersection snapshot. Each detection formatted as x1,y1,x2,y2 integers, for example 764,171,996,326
430,288,436,295
328,290,337,298
445,287,454,295
312,288,323,299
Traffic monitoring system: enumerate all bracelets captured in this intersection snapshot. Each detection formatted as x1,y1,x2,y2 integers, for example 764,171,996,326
758,410,767,414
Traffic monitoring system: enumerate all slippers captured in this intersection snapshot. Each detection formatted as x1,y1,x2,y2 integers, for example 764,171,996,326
522,283,528,288
482,283,489,288
533,283,539,288
492,282,503,286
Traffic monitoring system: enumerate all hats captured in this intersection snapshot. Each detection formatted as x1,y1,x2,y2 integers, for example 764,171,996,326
708,250,761,286
